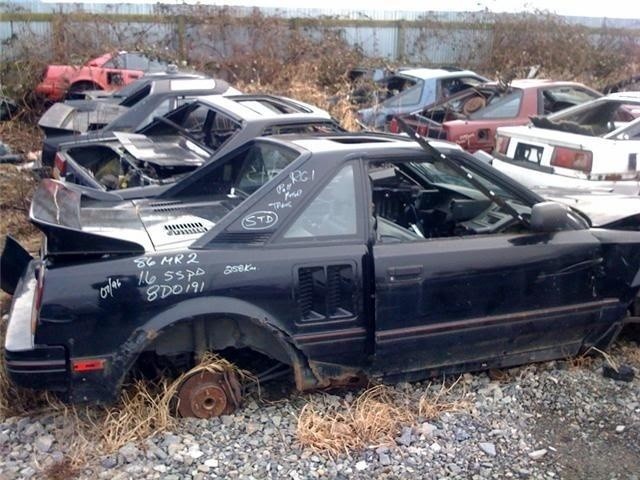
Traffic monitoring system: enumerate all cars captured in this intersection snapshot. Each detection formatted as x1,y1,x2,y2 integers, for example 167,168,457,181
30,51,640,198
1,113,639,419
